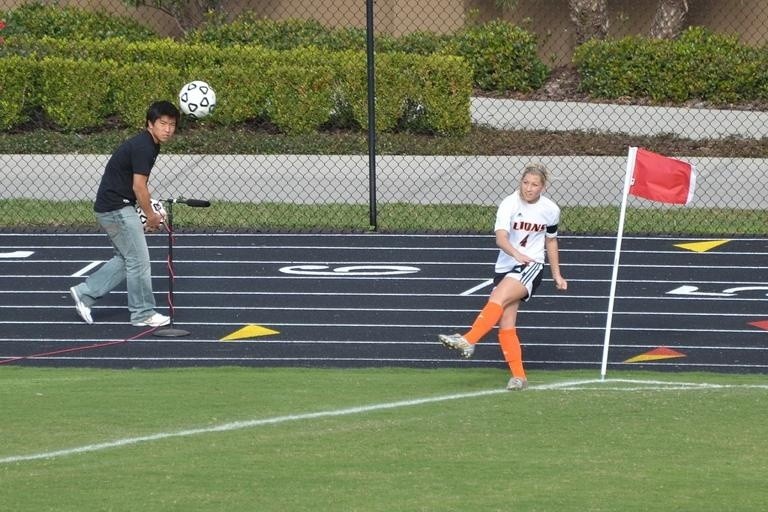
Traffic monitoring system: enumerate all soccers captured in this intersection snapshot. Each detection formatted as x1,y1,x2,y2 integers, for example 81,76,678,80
135,198,168,232
179,81,217,120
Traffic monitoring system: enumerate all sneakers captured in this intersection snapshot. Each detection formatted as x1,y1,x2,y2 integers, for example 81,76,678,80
506,377,527,391
437,333,475,360
69,285,93,326
132,313,170,327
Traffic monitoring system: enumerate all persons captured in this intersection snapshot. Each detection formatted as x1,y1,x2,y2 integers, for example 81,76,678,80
68,100,181,326
438,163,568,392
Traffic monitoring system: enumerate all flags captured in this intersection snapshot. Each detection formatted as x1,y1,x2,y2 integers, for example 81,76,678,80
626,147,697,206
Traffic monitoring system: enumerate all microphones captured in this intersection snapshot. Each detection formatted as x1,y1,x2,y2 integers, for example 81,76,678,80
158,196,210,209
